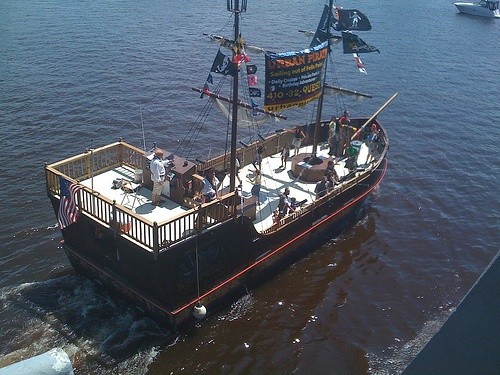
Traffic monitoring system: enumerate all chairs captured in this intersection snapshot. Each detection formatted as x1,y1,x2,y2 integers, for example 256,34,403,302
119,177,149,211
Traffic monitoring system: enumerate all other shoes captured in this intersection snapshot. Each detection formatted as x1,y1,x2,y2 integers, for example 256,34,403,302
158,200,165,203
279,166,282,168
283,167,286,170
152,201,160,206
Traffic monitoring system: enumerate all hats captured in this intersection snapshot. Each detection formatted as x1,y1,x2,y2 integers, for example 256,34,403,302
371,124,377,129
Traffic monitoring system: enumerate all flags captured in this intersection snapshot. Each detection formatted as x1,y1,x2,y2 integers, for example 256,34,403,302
321,6,381,75
263,39,329,110
200,46,261,107
57,176,85,229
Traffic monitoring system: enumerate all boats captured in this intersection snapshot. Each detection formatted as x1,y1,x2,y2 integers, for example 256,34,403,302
453,0,500,18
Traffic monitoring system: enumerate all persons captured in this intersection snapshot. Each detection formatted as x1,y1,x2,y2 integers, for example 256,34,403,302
150,150,175,205
210,111,380,217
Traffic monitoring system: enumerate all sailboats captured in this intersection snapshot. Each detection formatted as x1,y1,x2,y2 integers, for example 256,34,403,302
43,0,390,333
